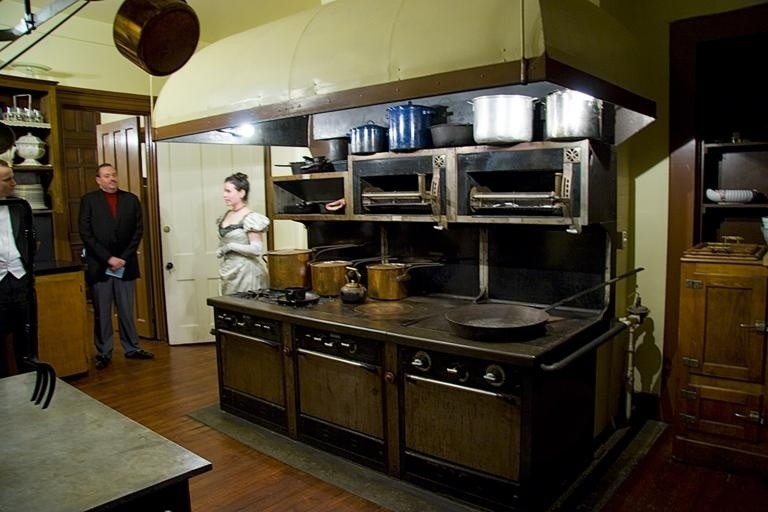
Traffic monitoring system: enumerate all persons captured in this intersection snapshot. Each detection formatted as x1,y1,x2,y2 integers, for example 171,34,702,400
0,158,37,378
77,163,154,369
214,172,270,296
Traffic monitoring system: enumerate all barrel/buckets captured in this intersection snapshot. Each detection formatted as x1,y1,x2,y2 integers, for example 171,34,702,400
384,100,454,152
467,95,541,145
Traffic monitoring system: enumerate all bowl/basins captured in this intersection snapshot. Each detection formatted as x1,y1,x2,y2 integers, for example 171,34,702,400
704,187,755,206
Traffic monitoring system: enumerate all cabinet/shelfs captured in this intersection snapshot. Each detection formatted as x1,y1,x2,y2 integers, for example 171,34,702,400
34,270,97,379
660,8,768,475
0,74,71,273
263,139,592,230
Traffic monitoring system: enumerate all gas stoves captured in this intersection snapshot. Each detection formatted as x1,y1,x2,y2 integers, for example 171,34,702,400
227,286,340,311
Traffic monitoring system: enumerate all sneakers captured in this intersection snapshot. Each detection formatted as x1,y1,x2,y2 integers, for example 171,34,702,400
124,349,154,360
95,354,111,368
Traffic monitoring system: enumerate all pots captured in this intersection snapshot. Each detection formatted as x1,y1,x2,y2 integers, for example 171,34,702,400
445,267,646,342
346,120,387,155
302,156,338,172
261,242,445,305
308,136,352,161
209,306,526,512
273,162,306,173
545,88,604,141
111,2,200,75
430,121,475,146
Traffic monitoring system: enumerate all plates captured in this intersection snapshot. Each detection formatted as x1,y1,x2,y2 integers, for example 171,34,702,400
14,183,48,209
